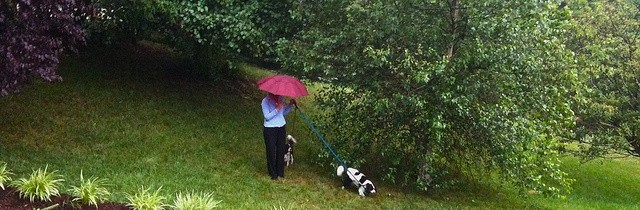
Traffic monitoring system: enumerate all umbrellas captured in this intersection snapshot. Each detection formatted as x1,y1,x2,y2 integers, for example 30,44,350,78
257,76,309,103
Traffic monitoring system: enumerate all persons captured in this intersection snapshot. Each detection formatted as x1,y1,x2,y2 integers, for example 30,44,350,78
261,92,294,180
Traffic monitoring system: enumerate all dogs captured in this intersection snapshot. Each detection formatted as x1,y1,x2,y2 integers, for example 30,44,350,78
337,161,377,199
280,135,296,167
